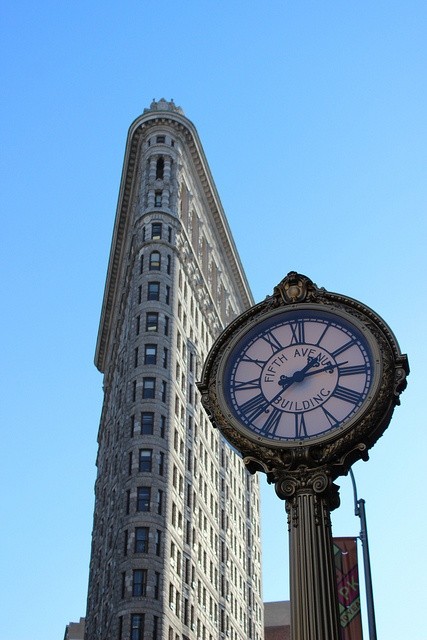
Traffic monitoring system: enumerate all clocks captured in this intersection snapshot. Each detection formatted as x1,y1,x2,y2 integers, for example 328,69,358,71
196,271,410,481
